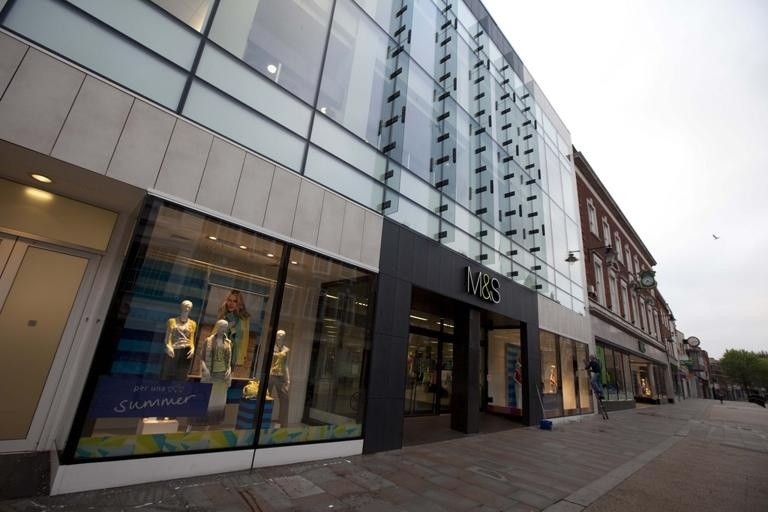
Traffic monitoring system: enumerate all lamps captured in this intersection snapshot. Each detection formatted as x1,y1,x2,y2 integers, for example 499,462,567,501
565,249,580,262
589,243,618,265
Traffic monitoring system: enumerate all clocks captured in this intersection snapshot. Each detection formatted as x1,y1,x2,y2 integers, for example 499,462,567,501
638,269,657,289
688,336,700,347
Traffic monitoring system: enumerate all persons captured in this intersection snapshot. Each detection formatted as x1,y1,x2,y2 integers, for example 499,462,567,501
719,388,725,404
550,365,557,392
513,362,523,409
217,290,251,379
165,300,197,379
407,351,416,389
201,319,233,424
267,329,291,429
585,354,605,399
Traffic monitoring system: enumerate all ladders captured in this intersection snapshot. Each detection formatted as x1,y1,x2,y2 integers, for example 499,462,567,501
582,358,610,419
